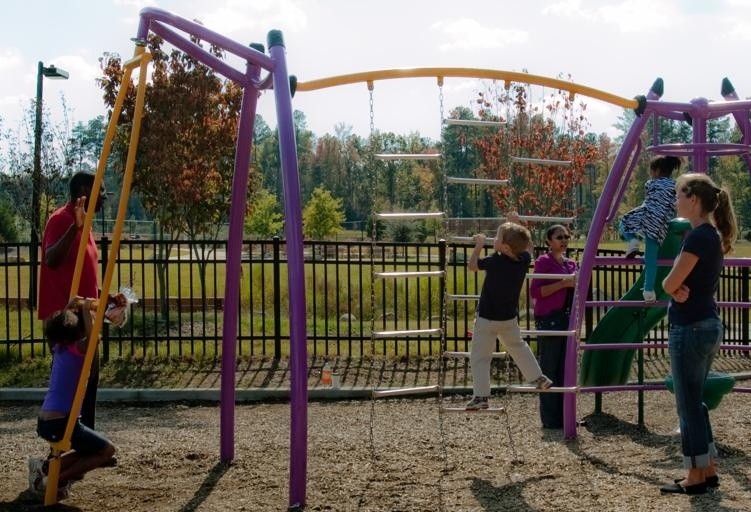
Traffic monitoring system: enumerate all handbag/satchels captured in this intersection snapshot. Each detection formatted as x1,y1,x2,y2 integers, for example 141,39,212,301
536,310,569,331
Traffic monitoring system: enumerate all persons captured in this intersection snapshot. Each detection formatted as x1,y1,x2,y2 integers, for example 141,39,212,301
527,224,580,430
462,220,555,411
659,171,740,497
25,292,116,504
616,151,682,303
35,171,129,430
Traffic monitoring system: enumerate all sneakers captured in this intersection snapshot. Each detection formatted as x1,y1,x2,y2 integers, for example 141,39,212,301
466,396,489,408
535,375,553,389
660,474,719,494
640,288,656,303
28,458,47,497
626,245,639,258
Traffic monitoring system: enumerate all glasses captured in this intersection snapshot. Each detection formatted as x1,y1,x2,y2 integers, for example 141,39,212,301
556,235,571,240
